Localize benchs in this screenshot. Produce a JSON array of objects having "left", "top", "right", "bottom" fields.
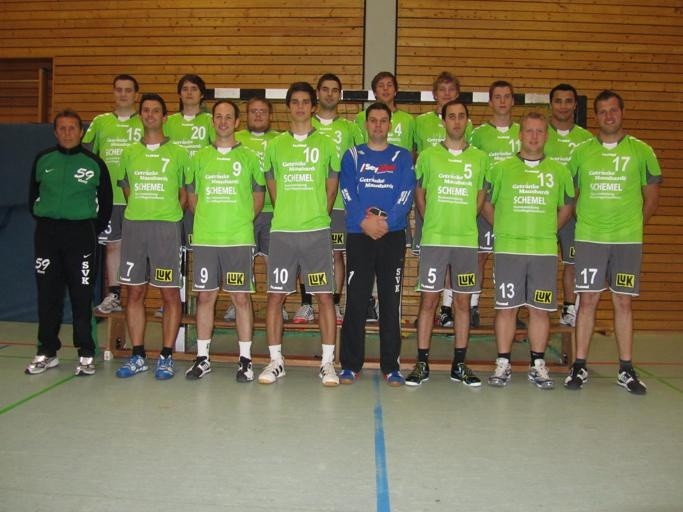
[{"left": 94, "top": 305, "right": 613, "bottom": 375}]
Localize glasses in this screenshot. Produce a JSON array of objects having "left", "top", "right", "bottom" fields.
[{"left": 248, "top": 108, "right": 269, "bottom": 116}]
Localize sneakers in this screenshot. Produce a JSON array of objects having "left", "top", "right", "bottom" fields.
[
  {"left": 338, "top": 369, "right": 361, "bottom": 384},
  {"left": 564, "top": 366, "right": 588, "bottom": 389},
  {"left": 258, "top": 356, "right": 286, "bottom": 383},
  {"left": 185, "top": 356, "right": 211, "bottom": 379},
  {"left": 293, "top": 305, "right": 314, "bottom": 324},
  {"left": 470, "top": 307, "right": 480, "bottom": 329},
  {"left": 405, "top": 360, "right": 429, "bottom": 386},
  {"left": 223, "top": 305, "right": 235, "bottom": 323},
  {"left": 451, "top": 363, "right": 482, "bottom": 386},
  {"left": 94, "top": 293, "right": 124, "bottom": 313},
  {"left": 25, "top": 353, "right": 59, "bottom": 374},
  {"left": 366, "top": 296, "right": 378, "bottom": 322},
  {"left": 155, "top": 354, "right": 174, "bottom": 379},
  {"left": 74, "top": 356, "right": 96, "bottom": 376},
  {"left": 236, "top": 357, "right": 254, "bottom": 382},
  {"left": 560, "top": 305, "right": 577, "bottom": 327},
  {"left": 335, "top": 304, "right": 344, "bottom": 325},
  {"left": 527, "top": 358, "right": 554, "bottom": 389},
  {"left": 318, "top": 362, "right": 339, "bottom": 387},
  {"left": 282, "top": 308, "right": 289, "bottom": 321},
  {"left": 116, "top": 354, "right": 148, "bottom": 378},
  {"left": 517, "top": 318, "right": 526, "bottom": 329},
  {"left": 154, "top": 306, "right": 164, "bottom": 318},
  {"left": 440, "top": 306, "right": 453, "bottom": 328},
  {"left": 616, "top": 369, "right": 647, "bottom": 394},
  {"left": 384, "top": 370, "right": 404, "bottom": 387},
  {"left": 487, "top": 358, "right": 511, "bottom": 386}
]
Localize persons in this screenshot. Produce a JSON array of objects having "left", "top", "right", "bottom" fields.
[
  {"left": 292, "top": 74, "right": 365, "bottom": 326},
  {"left": 540, "top": 82, "right": 595, "bottom": 328},
  {"left": 480, "top": 113, "right": 574, "bottom": 389},
  {"left": 466, "top": 79, "right": 527, "bottom": 335},
  {"left": 79, "top": 74, "right": 144, "bottom": 317},
  {"left": 413, "top": 70, "right": 474, "bottom": 330},
  {"left": 562, "top": 90, "right": 662, "bottom": 394},
  {"left": 223, "top": 96, "right": 289, "bottom": 322},
  {"left": 354, "top": 70, "right": 413, "bottom": 323},
  {"left": 115, "top": 92, "right": 191, "bottom": 380},
  {"left": 154, "top": 75, "right": 217, "bottom": 318},
  {"left": 403, "top": 101, "right": 491, "bottom": 388},
  {"left": 23, "top": 108, "right": 112, "bottom": 376},
  {"left": 256, "top": 81, "right": 340, "bottom": 387},
  {"left": 337, "top": 103, "right": 416, "bottom": 388},
  {"left": 185, "top": 99, "right": 266, "bottom": 382}
]
